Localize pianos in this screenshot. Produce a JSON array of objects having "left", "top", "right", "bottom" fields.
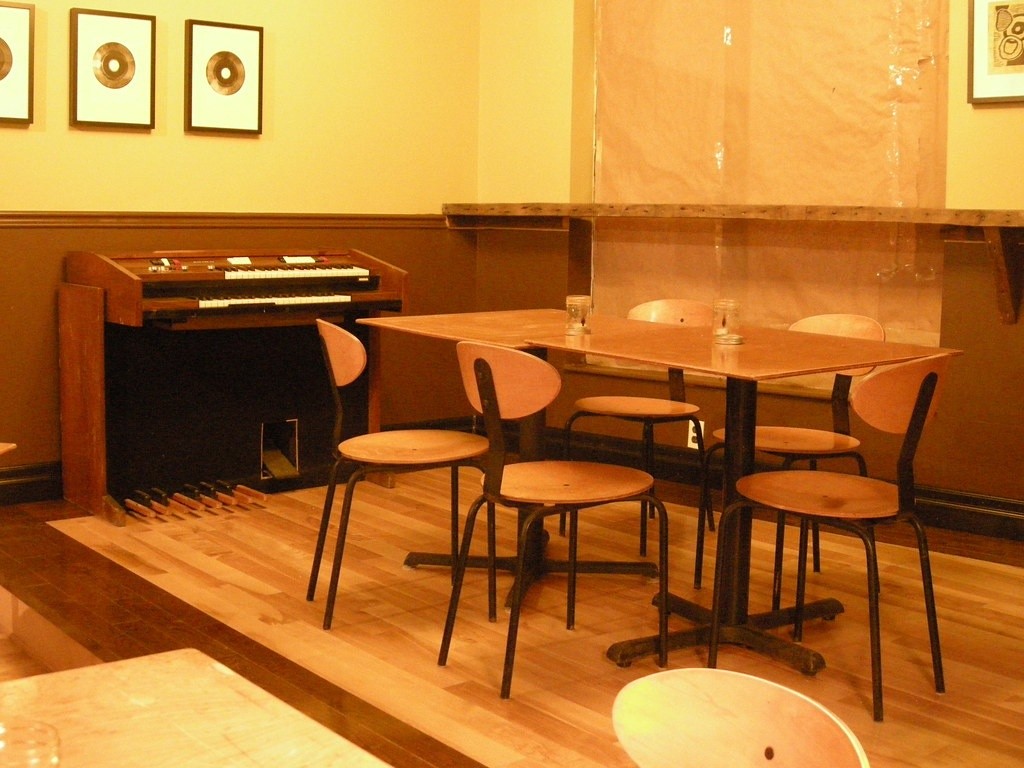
[{"left": 51, "top": 246, "right": 411, "bottom": 528}]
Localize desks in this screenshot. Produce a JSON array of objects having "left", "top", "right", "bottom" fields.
[
  {"left": 0, "top": 648, "right": 394, "bottom": 768},
  {"left": 359, "top": 308, "right": 968, "bottom": 676}
]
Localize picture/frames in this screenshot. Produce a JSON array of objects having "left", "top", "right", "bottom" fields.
[
  {"left": 0, "top": 1, "right": 36, "bottom": 124},
  {"left": 184, "top": 18, "right": 264, "bottom": 135},
  {"left": 69, "top": 8, "right": 157, "bottom": 129},
  {"left": 967, "top": 0, "right": 1024, "bottom": 103}
]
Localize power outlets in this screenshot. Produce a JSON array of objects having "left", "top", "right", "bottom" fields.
[{"left": 687, "top": 420, "right": 705, "bottom": 449}]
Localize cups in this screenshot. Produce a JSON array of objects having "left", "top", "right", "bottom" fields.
[
  {"left": 712, "top": 299, "right": 744, "bottom": 345},
  {"left": 564, "top": 295, "right": 591, "bottom": 336}
]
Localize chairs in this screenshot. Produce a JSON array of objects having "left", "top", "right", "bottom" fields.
[
  {"left": 611, "top": 668, "right": 871, "bottom": 768},
  {"left": 559, "top": 298, "right": 718, "bottom": 557},
  {"left": 437, "top": 341, "right": 670, "bottom": 699},
  {"left": 306, "top": 318, "right": 498, "bottom": 630},
  {"left": 694, "top": 313, "right": 886, "bottom": 612},
  {"left": 706, "top": 353, "right": 953, "bottom": 724}
]
